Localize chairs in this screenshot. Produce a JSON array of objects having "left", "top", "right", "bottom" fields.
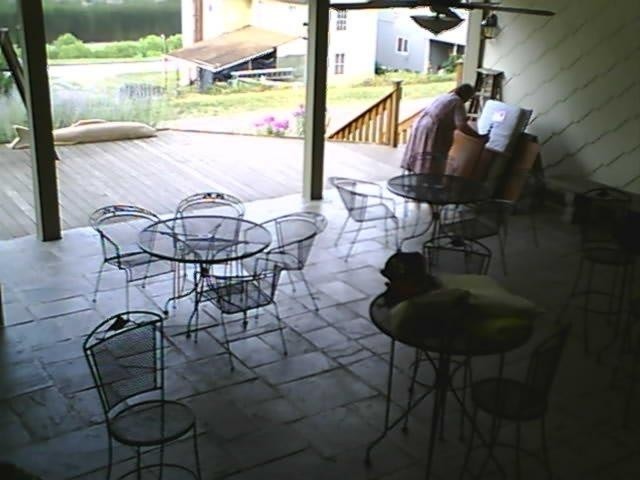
[
  {"left": 607, "top": 219, "right": 640, "bottom": 408},
  {"left": 422, "top": 235, "right": 493, "bottom": 276},
  {"left": 136, "top": 215, "right": 273, "bottom": 343},
  {"left": 83, "top": 310, "right": 202, "bottom": 479},
  {"left": 438, "top": 196, "right": 514, "bottom": 278},
  {"left": 471, "top": 319, "right": 574, "bottom": 478},
  {"left": 88, "top": 204, "right": 178, "bottom": 321},
  {"left": 170, "top": 191, "right": 246, "bottom": 311},
  {"left": 241, "top": 210, "right": 329, "bottom": 319},
  {"left": 194, "top": 257, "right": 290, "bottom": 374},
  {"left": 553, "top": 187, "right": 632, "bottom": 359},
  {"left": 327, "top": 176, "right": 399, "bottom": 264}
]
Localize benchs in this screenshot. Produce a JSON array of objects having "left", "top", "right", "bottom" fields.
[{"left": 545, "top": 176, "right": 639, "bottom": 226}]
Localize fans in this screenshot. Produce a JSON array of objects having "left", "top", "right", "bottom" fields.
[{"left": 329, "top": 0, "right": 555, "bottom": 35}]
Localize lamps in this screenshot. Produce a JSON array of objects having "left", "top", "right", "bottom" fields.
[{"left": 480, "top": 14, "right": 496, "bottom": 39}]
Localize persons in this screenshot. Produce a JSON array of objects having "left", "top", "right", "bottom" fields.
[{"left": 400, "top": 83, "right": 489, "bottom": 177}]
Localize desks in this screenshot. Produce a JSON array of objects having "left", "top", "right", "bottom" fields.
[
  {"left": 387, "top": 173, "right": 490, "bottom": 253},
  {"left": 365, "top": 276, "right": 538, "bottom": 478}
]
